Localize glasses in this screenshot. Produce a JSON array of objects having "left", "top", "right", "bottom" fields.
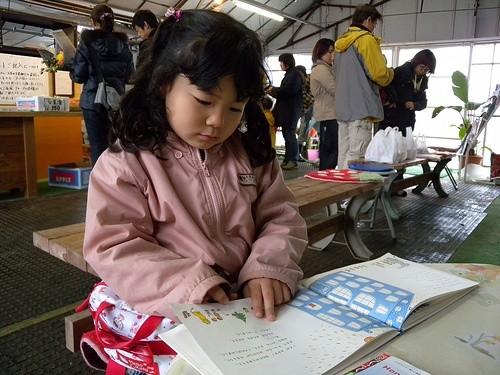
[{"left": 420, "top": 64, "right": 430, "bottom": 73}]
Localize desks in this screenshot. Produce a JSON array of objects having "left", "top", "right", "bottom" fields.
[
  {"left": 0, "top": 112, "right": 86, "bottom": 199},
  {"left": 161, "top": 263, "right": 500, "bottom": 375}
]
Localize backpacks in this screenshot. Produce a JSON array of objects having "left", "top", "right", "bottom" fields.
[{"left": 74, "top": 280, "right": 178, "bottom": 374}]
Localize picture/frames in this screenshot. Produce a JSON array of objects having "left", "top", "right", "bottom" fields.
[{"left": 52, "top": 67, "right": 74, "bottom": 98}]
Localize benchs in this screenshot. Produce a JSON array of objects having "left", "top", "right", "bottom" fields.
[
  {"left": 32, "top": 168, "right": 389, "bottom": 283},
  {"left": 348, "top": 147, "right": 457, "bottom": 198}
]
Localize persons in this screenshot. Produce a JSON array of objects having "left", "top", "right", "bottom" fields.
[
  {"left": 334, "top": 3, "right": 395, "bottom": 210},
  {"left": 125, "top": 10, "right": 160, "bottom": 82},
  {"left": 260, "top": 97, "right": 278, "bottom": 150},
  {"left": 378, "top": 48, "right": 436, "bottom": 197},
  {"left": 263, "top": 52, "right": 303, "bottom": 169},
  {"left": 310, "top": 38, "right": 339, "bottom": 172},
  {"left": 69, "top": 3, "right": 135, "bottom": 168},
  {"left": 73, "top": 8, "right": 310, "bottom": 375},
  {"left": 295, "top": 65, "right": 321, "bottom": 162}
]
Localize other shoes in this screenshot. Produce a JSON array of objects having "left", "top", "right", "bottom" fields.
[
  {"left": 283, "top": 160, "right": 299, "bottom": 169},
  {"left": 392, "top": 189, "right": 407, "bottom": 197},
  {"left": 298, "top": 154, "right": 307, "bottom": 162},
  {"left": 280, "top": 161, "right": 287, "bottom": 167}
]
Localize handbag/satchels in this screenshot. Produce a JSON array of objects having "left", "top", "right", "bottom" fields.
[{"left": 94, "top": 80, "right": 123, "bottom": 124}]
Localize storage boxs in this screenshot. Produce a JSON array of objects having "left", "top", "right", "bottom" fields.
[
  {"left": 47, "top": 164, "right": 93, "bottom": 190},
  {"left": 17, "top": 96, "right": 70, "bottom": 112}
]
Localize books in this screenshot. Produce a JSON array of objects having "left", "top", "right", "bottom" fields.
[{"left": 159, "top": 252, "right": 479, "bottom": 375}]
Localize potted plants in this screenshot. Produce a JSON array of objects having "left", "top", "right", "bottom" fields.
[{"left": 432, "top": 71, "right": 493, "bottom": 169}]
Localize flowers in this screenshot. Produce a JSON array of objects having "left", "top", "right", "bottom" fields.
[{"left": 37, "top": 48, "right": 65, "bottom": 75}]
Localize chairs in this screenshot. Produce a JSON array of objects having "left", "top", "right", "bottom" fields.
[{"left": 426, "top": 124, "right": 472, "bottom": 190}]
